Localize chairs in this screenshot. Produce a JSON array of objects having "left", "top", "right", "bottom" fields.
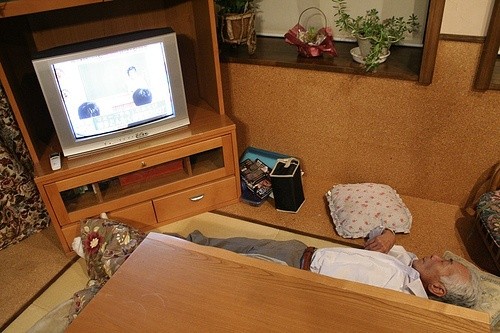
[{"left": 465, "top": 165, "right": 500, "bottom": 269}]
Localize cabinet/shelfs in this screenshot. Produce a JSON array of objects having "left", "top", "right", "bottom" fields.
[{"left": 0, "top": 0, "right": 241, "bottom": 256}]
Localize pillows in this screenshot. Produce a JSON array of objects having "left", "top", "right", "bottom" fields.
[
  {"left": 324, "top": 183, "right": 413, "bottom": 240},
  {"left": 441, "top": 251, "right": 500, "bottom": 327},
  {"left": 81, "top": 219, "right": 145, "bottom": 285}
]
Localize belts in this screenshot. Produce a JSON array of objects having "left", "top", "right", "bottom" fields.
[{"left": 300, "top": 246, "right": 316, "bottom": 271}]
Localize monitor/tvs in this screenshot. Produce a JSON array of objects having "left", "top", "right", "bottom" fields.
[{"left": 31, "top": 27, "right": 190, "bottom": 161}]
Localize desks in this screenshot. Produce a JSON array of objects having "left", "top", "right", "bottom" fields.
[{"left": 62, "top": 231, "right": 491, "bottom": 333}]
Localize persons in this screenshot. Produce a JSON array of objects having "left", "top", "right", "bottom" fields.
[
  {"left": 77, "top": 102, "right": 101, "bottom": 119},
  {"left": 128, "top": 67, "right": 141, "bottom": 88},
  {"left": 133, "top": 88, "right": 152, "bottom": 107},
  {"left": 72, "top": 212, "right": 481, "bottom": 310}
]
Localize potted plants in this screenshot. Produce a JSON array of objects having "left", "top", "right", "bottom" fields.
[
  {"left": 215, "top": 0, "right": 263, "bottom": 55},
  {"left": 333, "top": 0, "right": 422, "bottom": 72}
]
